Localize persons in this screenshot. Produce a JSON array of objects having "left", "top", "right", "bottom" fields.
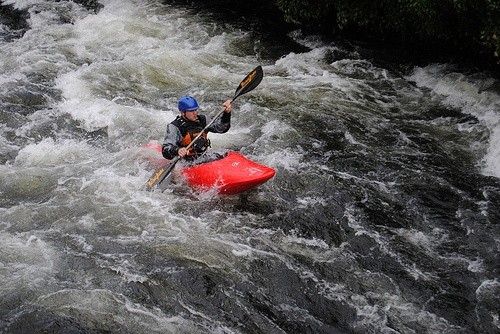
[{"left": 162, "top": 96, "right": 231, "bottom": 167}]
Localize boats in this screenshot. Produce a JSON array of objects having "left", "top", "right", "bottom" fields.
[{"left": 139, "top": 145, "right": 277, "bottom": 197}]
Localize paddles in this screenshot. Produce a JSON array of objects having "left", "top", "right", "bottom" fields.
[{"left": 146, "top": 65, "right": 264, "bottom": 192}]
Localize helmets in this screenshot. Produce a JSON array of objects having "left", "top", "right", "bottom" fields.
[{"left": 178, "top": 96, "right": 199, "bottom": 111}]
